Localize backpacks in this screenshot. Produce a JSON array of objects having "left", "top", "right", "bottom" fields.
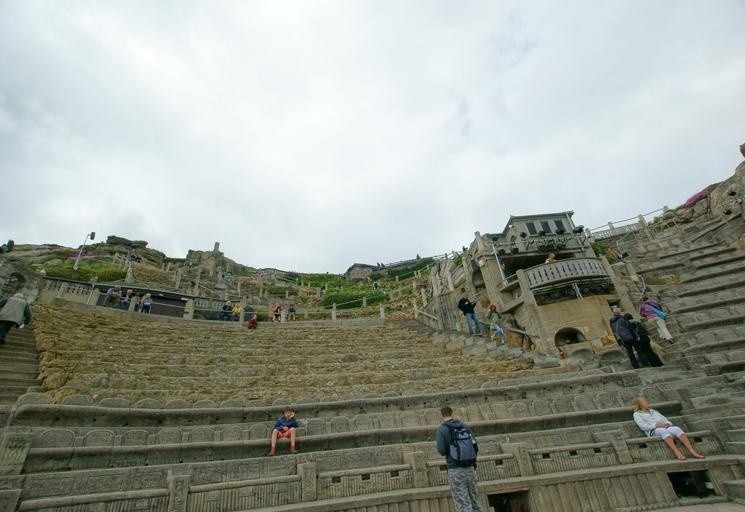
[{"left": 442, "top": 423, "right": 478, "bottom": 463}]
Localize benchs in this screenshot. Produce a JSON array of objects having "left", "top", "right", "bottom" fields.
[{"left": 7, "top": 302, "right": 745, "bottom": 512}]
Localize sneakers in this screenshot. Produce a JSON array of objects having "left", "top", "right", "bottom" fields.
[
  {"left": 268, "top": 448, "right": 275, "bottom": 455},
  {"left": 291, "top": 446, "right": 296, "bottom": 452}
]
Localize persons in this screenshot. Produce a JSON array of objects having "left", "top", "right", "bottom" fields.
[
  {"left": 609, "top": 307, "right": 650, "bottom": 369},
  {"left": 0, "top": 293, "right": 31, "bottom": 345},
  {"left": 119, "top": 288, "right": 132, "bottom": 310},
  {"left": 632, "top": 395, "right": 705, "bottom": 460},
  {"left": 268, "top": 406, "right": 299, "bottom": 456},
  {"left": 457, "top": 292, "right": 481, "bottom": 336},
  {"left": 624, "top": 312, "right": 664, "bottom": 368},
  {"left": 140, "top": 293, "right": 152, "bottom": 313},
  {"left": 486, "top": 304, "right": 505, "bottom": 343},
  {"left": 545, "top": 252, "right": 556, "bottom": 263},
  {"left": 436, "top": 407, "right": 487, "bottom": 512},
  {"left": 247, "top": 314, "right": 257, "bottom": 328},
  {"left": 271, "top": 302, "right": 282, "bottom": 322},
  {"left": 640, "top": 296, "right": 674, "bottom": 344},
  {"left": 101, "top": 285, "right": 122, "bottom": 308},
  {"left": 233, "top": 302, "right": 240, "bottom": 317},
  {"left": 288, "top": 303, "right": 296, "bottom": 320},
  {"left": 221, "top": 300, "right": 233, "bottom": 321}
]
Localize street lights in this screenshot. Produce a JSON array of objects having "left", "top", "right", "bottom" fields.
[{"left": 74, "top": 230, "right": 96, "bottom": 270}]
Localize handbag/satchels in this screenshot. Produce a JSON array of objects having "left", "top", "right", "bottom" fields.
[
  {"left": 646, "top": 304, "right": 668, "bottom": 319},
  {"left": 614, "top": 333, "right": 624, "bottom": 348}
]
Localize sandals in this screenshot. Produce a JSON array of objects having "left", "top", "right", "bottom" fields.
[{"left": 677, "top": 454, "right": 704, "bottom": 459}]
